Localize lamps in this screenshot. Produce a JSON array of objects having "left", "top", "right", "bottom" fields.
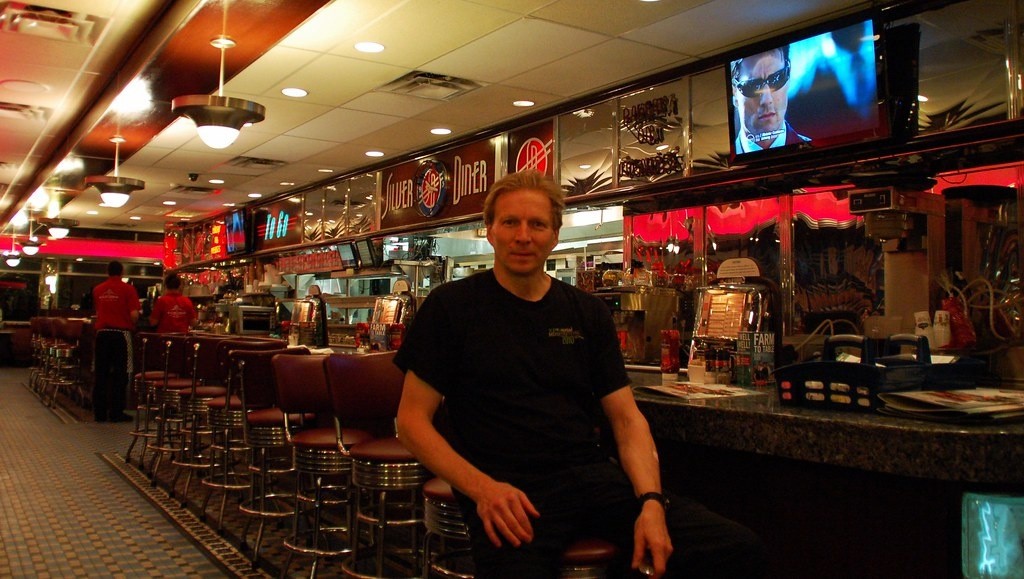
[
  {"left": 2, "top": 236, "right": 22, "bottom": 267},
  {"left": 171, "top": 0, "right": 266, "bottom": 150},
  {"left": 84, "top": 138, "right": 144, "bottom": 208},
  {"left": 37, "top": 190, "right": 79, "bottom": 238},
  {"left": 20, "top": 218, "right": 44, "bottom": 255}
]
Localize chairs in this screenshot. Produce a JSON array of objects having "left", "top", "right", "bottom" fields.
[{"left": 771, "top": 333, "right": 984, "bottom": 414}]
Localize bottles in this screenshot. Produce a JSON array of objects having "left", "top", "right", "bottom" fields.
[{"left": 660, "top": 329, "right": 680, "bottom": 386}]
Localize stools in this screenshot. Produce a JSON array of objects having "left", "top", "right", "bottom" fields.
[{"left": 28, "top": 317, "right": 665, "bottom": 579}]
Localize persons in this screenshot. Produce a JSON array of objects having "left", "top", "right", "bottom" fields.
[
  {"left": 148, "top": 273, "right": 197, "bottom": 335},
  {"left": 730, "top": 44, "right": 811, "bottom": 155},
  {"left": 393, "top": 171, "right": 764, "bottom": 579},
  {"left": 92, "top": 261, "right": 141, "bottom": 422}
]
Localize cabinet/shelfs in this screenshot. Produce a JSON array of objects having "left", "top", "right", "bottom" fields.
[{"left": 318, "top": 269, "right": 394, "bottom": 349}]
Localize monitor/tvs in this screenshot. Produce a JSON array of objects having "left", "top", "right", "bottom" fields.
[
  {"left": 355, "top": 238, "right": 376, "bottom": 267},
  {"left": 338, "top": 241, "right": 358, "bottom": 268},
  {"left": 224, "top": 208, "right": 248, "bottom": 256},
  {"left": 724, "top": 6, "right": 891, "bottom": 169}
]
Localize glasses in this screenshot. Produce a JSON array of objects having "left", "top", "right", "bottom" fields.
[{"left": 732, "top": 59, "right": 791, "bottom": 98}]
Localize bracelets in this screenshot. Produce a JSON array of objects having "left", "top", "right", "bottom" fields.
[{"left": 638, "top": 492, "right": 671, "bottom": 511}]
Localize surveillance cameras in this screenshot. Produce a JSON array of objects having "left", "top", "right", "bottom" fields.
[{"left": 189, "top": 173, "right": 198, "bottom": 181}]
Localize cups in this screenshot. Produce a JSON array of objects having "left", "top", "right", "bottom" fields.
[
  {"left": 914, "top": 310, "right": 952, "bottom": 350},
  {"left": 688, "top": 364, "right": 704, "bottom": 383}
]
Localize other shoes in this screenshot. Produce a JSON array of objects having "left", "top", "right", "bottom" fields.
[
  {"left": 94, "top": 412, "right": 108, "bottom": 420},
  {"left": 109, "top": 410, "right": 134, "bottom": 423}
]
